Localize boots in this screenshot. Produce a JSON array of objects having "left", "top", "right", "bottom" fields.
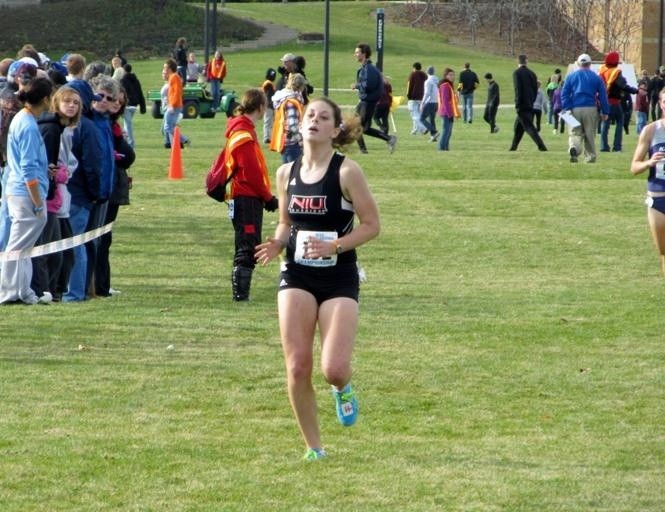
[{"left": 232, "top": 266, "right": 252, "bottom": 302}]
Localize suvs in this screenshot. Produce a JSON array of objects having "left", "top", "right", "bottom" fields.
[{"left": 147, "top": 80, "right": 248, "bottom": 118}]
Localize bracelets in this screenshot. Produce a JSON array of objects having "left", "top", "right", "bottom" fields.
[{"left": 332, "top": 238, "right": 343, "bottom": 255}]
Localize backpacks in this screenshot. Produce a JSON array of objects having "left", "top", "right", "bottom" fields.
[{"left": 207, "top": 147, "right": 238, "bottom": 202}]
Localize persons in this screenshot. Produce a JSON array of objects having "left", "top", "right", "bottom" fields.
[
  {"left": 631, "top": 88, "right": 665, "bottom": 275},
  {"left": 545, "top": 52, "right": 640, "bottom": 163},
  {"left": 252, "top": 98, "right": 381, "bottom": 466},
  {"left": 224, "top": 89, "right": 279, "bottom": 300},
  {"left": 635, "top": 65, "right": 665, "bottom": 136},
  {"left": 456, "top": 62, "right": 501, "bottom": 134},
  {"left": 160, "top": 35, "right": 314, "bottom": 164},
  {"left": 507, "top": 55, "right": 549, "bottom": 152},
  {"left": 407, "top": 62, "right": 458, "bottom": 151},
  {"left": 352, "top": 44, "right": 397, "bottom": 154},
  {"left": 1, "top": 42, "right": 146, "bottom": 306}
]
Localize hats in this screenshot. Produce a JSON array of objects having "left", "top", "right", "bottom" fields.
[
  {"left": 578, "top": 54, "right": 592, "bottom": 64},
  {"left": 605, "top": 52, "right": 619, "bottom": 65},
  {"left": 7, "top": 52, "right": 112, "bottom": 101},
  {"left": 427, "top": 66, "right": 433, "bottom": 75},
  {"left": 280, "top": 53, "right": 295, "bottom": 61}
]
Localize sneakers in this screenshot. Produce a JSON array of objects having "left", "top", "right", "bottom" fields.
[
  {"left": 165, "top": 141, "right": 187, "bottom": 148},
  {"left": 303, "top": 448, "right": 327, "bottom": 460},
  {"left": 411, "top": 129, "right": 440, "bottom": 143},
  {"left": 333, "top": 384, "right": 358, "bottom": 426},
  {"left": 570, "top": 148, "right": 578, "bottom": 162},
  {"left": 39, "top": 288, "right": 122, "bottom": 303},
  {"left": 265, "top": 139, "right": 271, "bottom": 143},
  {"left": 388, "top": 135, "right": 397, "bottom": 154},
  {"left": 211, "top": 107, "right": 221, "bottom": 112},
  {"left": 360, "top": 150, "right": 368, "bottom": 155}
]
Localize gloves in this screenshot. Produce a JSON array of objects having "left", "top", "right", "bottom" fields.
[{"left": 265, "top": 196, "right": 278, "bottom": 212}]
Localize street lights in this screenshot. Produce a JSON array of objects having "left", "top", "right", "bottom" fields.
[{"left": 376, "top": 7, "right": 385, "bottom": 73}]
